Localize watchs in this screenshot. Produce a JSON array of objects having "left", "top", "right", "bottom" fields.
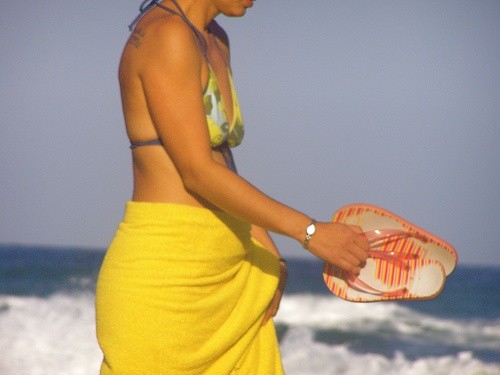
[{"left": 303, "top": 217, "right": 317, "bottom": 250}]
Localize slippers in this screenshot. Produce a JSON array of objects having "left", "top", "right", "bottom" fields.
[
  {"left": 330, "top": 204, "right": 458, "bottom": 276},
  {"left": 323, "top": 249, "right": 447, "bottom": 303}
]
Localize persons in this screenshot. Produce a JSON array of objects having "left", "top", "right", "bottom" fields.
[{"left": 95, "top": 0, "right": 368, "bottom": 375}]
